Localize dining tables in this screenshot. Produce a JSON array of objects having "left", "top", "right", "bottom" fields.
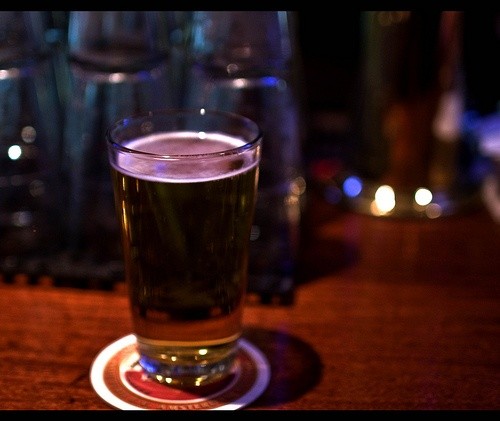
[{"left": 0, "top": 111, "right": 500, "bottom": 412}]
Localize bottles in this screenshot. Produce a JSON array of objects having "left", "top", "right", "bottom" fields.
[
  {"left": 180, "top": 8, "right": 305, "bottom": 303},
  {"left": 57, "top": 13, "right": 183, "bottom": 288},
  {"left": 3, "top": 12, "right": 71, "bottom": 288}
]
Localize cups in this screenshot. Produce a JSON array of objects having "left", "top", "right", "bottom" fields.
[
  {"left": 108, "top": 105, "right": 264, "bottom": 391},
  {"left": 325, "top": 11, "right": 486, "bottom": 223}
]
